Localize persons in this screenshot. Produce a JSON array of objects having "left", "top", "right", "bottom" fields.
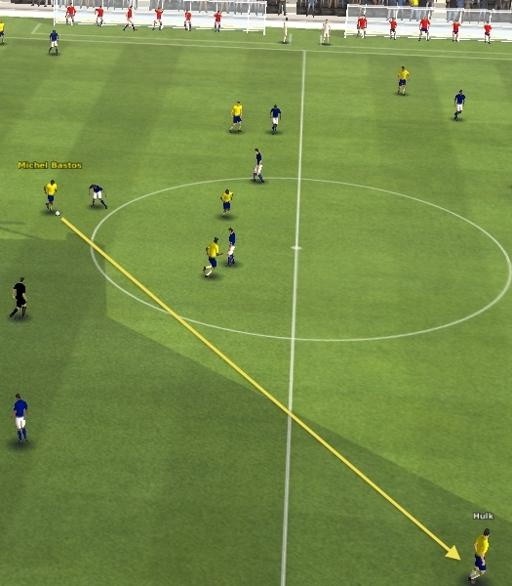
[
  {"left": 469, "top": 528, "right": 489, "bottom": 584},
  {"left": 12, "top": 393, "right": 28, "bottom": 443},
  {"left": 10, "top": 276, "right": 29, "bottom": 320},
  {"left": 1, "top": 0, "right": 512, "bottom": 586}
]
